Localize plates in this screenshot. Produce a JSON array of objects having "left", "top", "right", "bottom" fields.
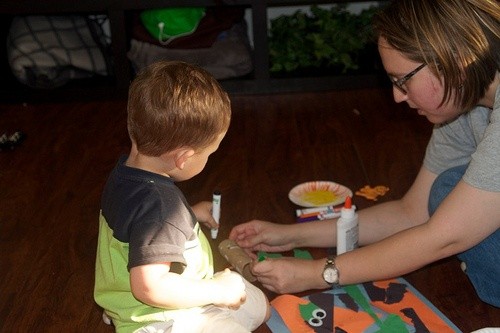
[{"left": 288, "top": 181, "right": 354, "bottom": 207}]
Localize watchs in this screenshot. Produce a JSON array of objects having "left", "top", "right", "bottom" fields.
[{"left": 321, "top": 255, "right": 342, "bottom": 289}]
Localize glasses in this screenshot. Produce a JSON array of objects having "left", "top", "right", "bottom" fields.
[{"left": 392, "top": 59, "right": 431, "bottom": 95}]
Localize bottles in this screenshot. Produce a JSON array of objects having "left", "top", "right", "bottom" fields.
[{"left": 336, "top": 196, "right": 360, "bottom": 256}]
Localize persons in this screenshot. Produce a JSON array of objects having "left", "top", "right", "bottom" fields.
[
  {"left": 92, "top": 59, "right": 272, "bottom": 333},
  {"left": 227, "top": 0, "right": 500, "bottom": 308}
]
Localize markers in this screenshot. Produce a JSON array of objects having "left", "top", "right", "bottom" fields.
[
  {"left": 285, "top": 201, "right": 359, "bottom": 226},
  {"left": 211, "top": 190, "right": 221, "bottom": 240}
]
